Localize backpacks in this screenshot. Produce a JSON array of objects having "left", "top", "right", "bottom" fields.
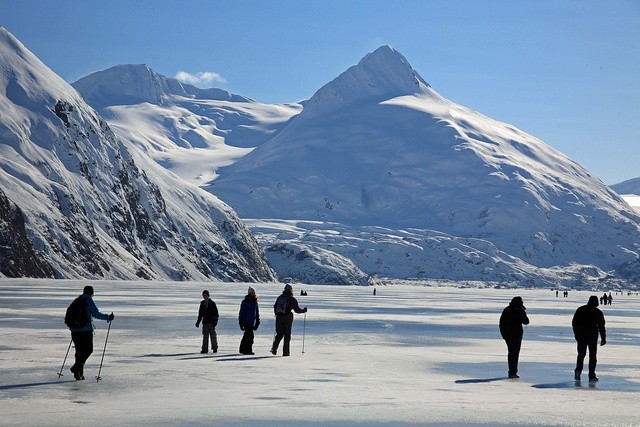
[
  {"left": 276, "top": 296, "right": 291, "bottom": 315},
  {"left": 65, "top": 295, "right": 90, "bottom": 330}
]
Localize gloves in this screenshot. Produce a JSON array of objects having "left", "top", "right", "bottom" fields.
[
  {"left": 109, "top": 313, "right": 114, "bottom": 320},
  {"left": 213, "top": 318, "right": 217, "bottom": 325},
  {"left": 239, "top": 321, "right": 244, "bottom": 331},
  {"left": 195, "top": 320, "right": 200, "bottom": 327},
  {"left": 303, "top": 307, "right": 307, "bottom": 312},
  {"left": 600, "top": 338, "right": 606, "bottom": 345},
  {"left": 253, "top": 322, "right": 260, "bottom": 330}
]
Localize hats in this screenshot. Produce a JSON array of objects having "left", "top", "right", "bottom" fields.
[
  {"left": 285, "top": 284, "right": 293, "bottom": 292},
  {"left": 203, "top": 290, "right": 209, "bottom": 297},
  {"left": 248, "top": 287, "right": 255, "bottom": 294},
  {"left": 85, "top": 286, "right": 94, "bottom": 295},
  {"left": 588, "top": 296, "right": 598, "bottom": 306}
]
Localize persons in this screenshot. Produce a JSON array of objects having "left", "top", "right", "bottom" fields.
[
  {"left": 498, "top": 296, "right": 530, "bottom": 378},
  {"left": 563, "top": 290, "right": 568, "bottom": 297},
  {"left": 600, "top": 292, "right": 614, "bottom": 305},
  {"left": 238, "top": 287, "right": 260, "bottom": 355},
  {"left": 195, "top": 290, "right": 219, "bottom": 353},
  {"left": 270, "top": 284, "right": 307, "bottom": 356},
  {"left": 571, "top": 296, "right": 607, "bottom": 381},
  {"left": 64, "top": 285, "right": 114, "bottom": 379},
  {"left": 556, "top": 290, "right": 559, "bottom": 296}
]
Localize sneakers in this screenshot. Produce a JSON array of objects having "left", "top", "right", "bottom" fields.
[
  {"left": 70, "top": 365, "right": 81, "bottom": 380},
  {"left": 213, "top": 349, "right": 217, "bottom": 353},
  {"left": 509, "top": 374, "right": 519, "bottom": 378},
  {"left": 271, "top": 348, "right": 277, "bottom": 355},
  {"left": 201, "top": 350, "right": 208, "bottom": 353},
  {"left": 80, "top": 375, "right": 84, "bottom": 379},
  {"left": 575, "top": 370, "right": 581, "bottom": 380},
  {"left": 590, "top": 376, "right": 598, "bottom": 381},
  {"left": 243, "top": 351, "right": 254, "bottom": 355}
]
